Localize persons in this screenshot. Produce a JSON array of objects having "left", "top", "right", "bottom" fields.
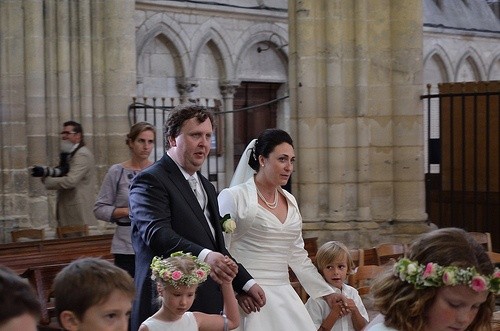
[
  {"left": 213, "top": 128, "right": 352, "bottom": 330},
  {"left": 300, "top": 238, "right": 369, "bottom": 331},
  {"left": 126, "top": 105, "right": 266, "bottom": 330},
  {"left": 39, "top": 121, "right": 99, "bottom": 240},
  {"left": 51, "top": 253, "right": 139, "bottom": 331},
  {"left": 93, "top": 120, "right": 157, "bottom": 284},
  {"left": 136, "top": 250, "right": 242, "bottom": 331},
  {"left": 361, "top": 224, "right": 500, "bottom": 331},
  {"left": 0, "top": 260, "right": 44, "bottom": 331}
]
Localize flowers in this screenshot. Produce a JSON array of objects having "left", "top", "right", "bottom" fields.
[
  {"left": 149, "top": 249, "right": 213, "bottom": 289},
  {"left": 394, "top": 257, "right": 499, "bottom": 294},
  {"left": 222, "top": 214, "right": 237, "bottom": 234}
]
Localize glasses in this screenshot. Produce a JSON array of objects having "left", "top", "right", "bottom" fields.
[{"left": 61, "top": 131, "right": 76, "bottom": 135}]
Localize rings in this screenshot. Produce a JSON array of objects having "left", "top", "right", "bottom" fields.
[{"left": 247, "top": 306, "right": 251, "bottom": 309}]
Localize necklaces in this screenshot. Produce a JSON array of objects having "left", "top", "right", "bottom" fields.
[{"left": 252, "top": 177, "right": 281, "bottom": 211}]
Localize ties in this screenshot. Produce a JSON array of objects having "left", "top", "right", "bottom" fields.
[{"left": 188, "top": 177, "right": 198, "bottom": 197}]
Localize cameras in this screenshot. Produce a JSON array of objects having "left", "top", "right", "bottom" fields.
[{"left": 32, "top": 165, "right": 67, "bottom": 177}]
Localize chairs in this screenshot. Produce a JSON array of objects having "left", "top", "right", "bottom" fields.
[
  {"left": 11, "top": 227, "right": 44, "bottom": 242},
  {"left": 290, "top": 232, "right": 500, "bottom": 314},
  {"left": 56, "top": 223, "right": 89, "bottom": 238}
]
